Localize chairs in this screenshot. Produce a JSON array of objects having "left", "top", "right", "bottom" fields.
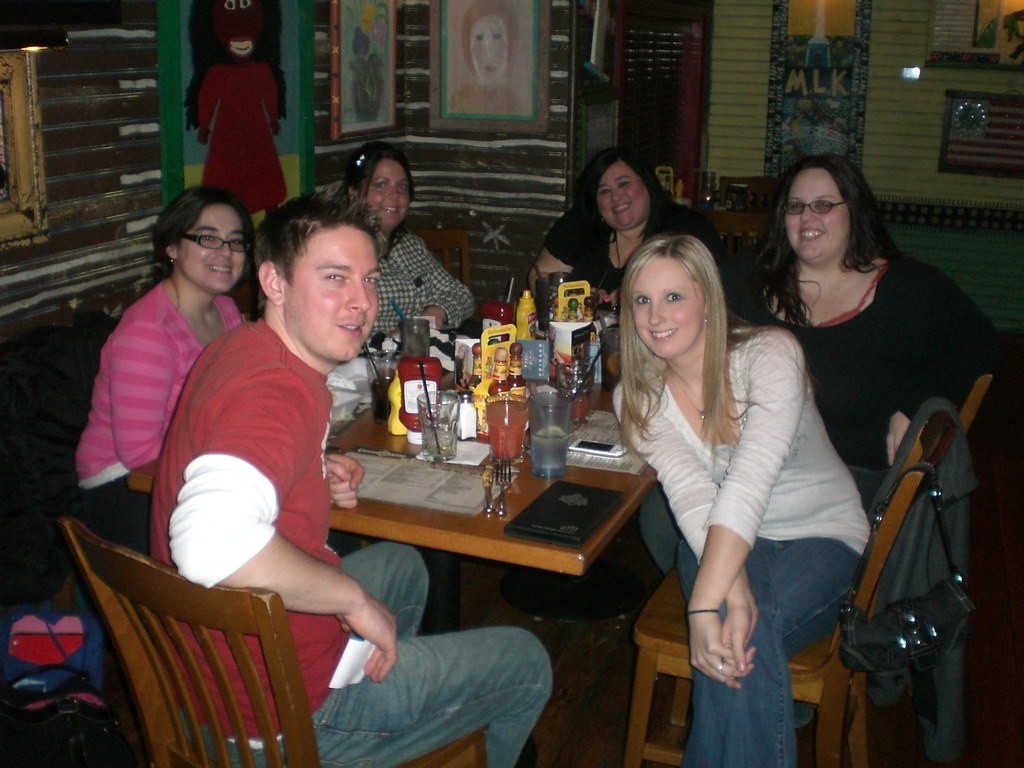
[
  {"left": 627, "top": 373, "right": 993, "bottom": 768},
  {"left": 410, "top": 228, "right": 471, "bottom": 292},
  {"left": 61, "top": 514, "right": 490, "bottom": 768},
  {"left": 695, "top": 176, "right": 782, "bottom": 263}
]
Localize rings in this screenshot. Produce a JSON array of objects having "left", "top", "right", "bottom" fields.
[{"left": 716, "top": 658, "right": 727, "bottom": 671}]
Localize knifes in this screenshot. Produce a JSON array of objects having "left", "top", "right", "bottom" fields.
[{"left": 482, "top": 465, "right": 494, "bottom": 513}]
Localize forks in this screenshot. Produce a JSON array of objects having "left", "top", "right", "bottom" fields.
[{"left": 496, "top": 459, "right": 511, "bottom": 519}]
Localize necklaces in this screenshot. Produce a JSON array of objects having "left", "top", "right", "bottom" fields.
[
  {"left": 611, "top": 229, "right": 643, "bottom": 267},
  {"left": 802, "top": 266, "right": 853, "bottom": 327},
  {"left": 667, "top": 365, "right": 706, "bottom": 421}
]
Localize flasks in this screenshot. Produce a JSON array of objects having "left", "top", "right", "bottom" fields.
[{"left": 542, "top": 272, "right": 571, "bottom": 332}]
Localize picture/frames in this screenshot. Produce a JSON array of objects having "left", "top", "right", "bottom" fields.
[
  {"left": 429, "top": 0, "right": 550, "bottom": 131},
  {"left": 925, "top": 0, "right": 1024, "bottom": 72},
  {"left": 155, "top": 0, "right": 315, "bottom": 231},
  {"left": 0, "top": 49, "right": 50, "bottom": 250},
  {"left": 937, "top": 89, "right": 1024, "bottom": 179},
  {"left": 330, "top": 0, "right": 397, "bottom": 141}
]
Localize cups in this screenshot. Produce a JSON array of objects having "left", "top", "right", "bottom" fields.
[
  {"left": 398, "top": 318, "right": 431, "bottom": 358},
  {"left": 417, "top": 391, "right": 461, "bottom": 462},
  {"left": 486, "top": 393, "right": 529, "bottom": 467},
  {"left": 529, "top": 391, "right": 573, "bottom": 479},
  {"left": 555, "top": 362, "right": 595, "bottom": 426},
  {"left": 367, "top": 350, "right": 403, "bottom": 425},
  {"left": 480, "top": 299, "right": 514, "bottom": 345},
  {"left": 599, "top": 327, "right": 621, "bottom": 390}
]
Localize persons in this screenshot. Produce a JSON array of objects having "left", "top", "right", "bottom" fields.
[
  {"left": 528, "top": 148, "right": 723, "bottom": 328},
  {"left": 637, "top": 149, "right": 1003, "bottom": 575},
  {"left": 74, "top": 184, "right": 363, "bottom": 558},
  {"left": 611, "top": 237, "right": 869, "bottom": 768},
  {"left": 150, "top": 205, "right": 553, "bottom": 768},
  {"left": 340, "top": 143, "right": 478, "bottom": 354}
]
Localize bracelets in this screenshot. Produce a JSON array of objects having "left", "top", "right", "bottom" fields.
[{"left": 685, "top": 608, "right": 721, "bottom": 614}]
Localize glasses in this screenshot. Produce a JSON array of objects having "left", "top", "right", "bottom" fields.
[
  {"left": 181, "top": 233, "right": 250, "bottom": 252},
  {"left": 781, "top": 199, "right": 850, "bottom": 216}
]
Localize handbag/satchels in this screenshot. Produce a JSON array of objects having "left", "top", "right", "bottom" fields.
[
  {"left": 0, "top": 605, "right": 108, "bottom": 716},
  {"left": 841, "top": 462, "right": 973, "bottom": 681}
]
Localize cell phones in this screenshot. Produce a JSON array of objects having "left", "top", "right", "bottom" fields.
[{"left": 568, "top": 439, "right": 628, "bottom": 457}]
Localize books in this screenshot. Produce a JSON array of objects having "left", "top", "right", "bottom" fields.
[{"left": 503, "top": 481, "right": 624, "bottom": 548}]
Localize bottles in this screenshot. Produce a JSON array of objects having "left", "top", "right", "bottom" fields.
[
  {"left": 488, "top": 347, "right": 510, "bottom": 396},
  {"left": 516, "top": 289, "right": 537, "bottom": 341},
  {"left": 387, "top": 370, "right": 408, "bottom": 436},
  {"left": 456, "top": 390, "right": 477, "bottom": 440},
  {"left": 399, "top": 356, "right": 442, "bottom": 445},
  {"left": 506, "top": 342, "right": 526, "bottom": 396},
  {"left": 467, "top": 343, "right": 482, "bottom": 392}
]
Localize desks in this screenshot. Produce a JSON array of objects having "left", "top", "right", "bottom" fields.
[{"left": 127, "top": 387, "right": 659, "bottom": 576}]
